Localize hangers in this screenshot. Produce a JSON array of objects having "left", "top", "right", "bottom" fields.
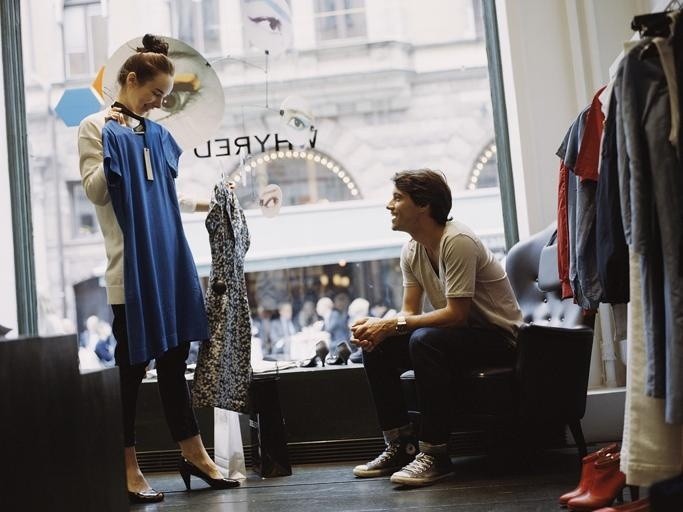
[
  {"left": 101, "top": 85, "right": 146, "bottom": 136},
  {"left": 630, "top": 0, "right": 683, "bottom": 38}
]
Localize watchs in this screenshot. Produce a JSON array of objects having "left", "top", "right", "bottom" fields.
[{"left": 395, "top": 316, "right": 407, "bottom": 336}]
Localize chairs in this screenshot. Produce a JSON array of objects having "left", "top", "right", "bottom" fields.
[{"left": 396, "top": 211, "right": 600, "bottom": 473}]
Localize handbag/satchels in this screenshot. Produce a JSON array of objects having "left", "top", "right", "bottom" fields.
[
  {"left": 248, "top": 366, "right": 292, "bottom": 478},
  {"left": 213, "top": 407, "right": 258, "bottom": 486}
]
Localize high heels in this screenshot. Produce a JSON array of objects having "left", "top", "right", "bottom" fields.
[
  {"left": 178, "top": 456, "right": 240, "bottom": 489},
  {"left": 299, "top": 340, "right": 329, "bottom": 367},
  {"left": 559, "top": 443, "right": 624, "bottom": 507},
  {"left": 127, "top": 488, "right": 164, "bottom": 504},
  {"left": 327, "top": 342, "right": 351, "bottom": 365},
  {"left": 567, "top": 453, "right": 641, "bottom": 510}
]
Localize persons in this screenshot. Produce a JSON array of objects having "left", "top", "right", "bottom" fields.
[
  {"left": 78, "top": 314, "right": 113, "bottom": 366},
  {"left": 251, "top": 297, "right": 388, "bottom": 365},
  {"left": 74, "top": 34, "right": 240, "bottom": 502},
  {"left": 349, "top": 167, "right": 527, "bottom": 487}
]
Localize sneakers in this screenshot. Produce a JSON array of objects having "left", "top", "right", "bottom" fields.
[
  {"left": 352, "top": 424, "right": 418, "bottom": 477},
  {"left": 390, "top": 441, "right": 456, "bottom": 487}
]
